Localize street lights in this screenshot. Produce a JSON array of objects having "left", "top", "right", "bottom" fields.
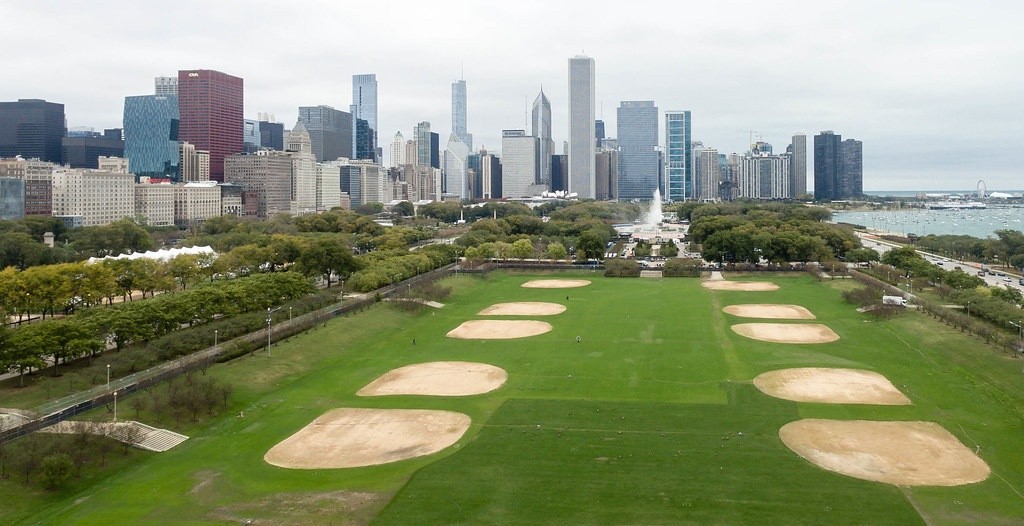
[
  {"left": 112, "top": 388, "right": 118, "bottom": 422},
  {"left": 289, "top": 306, "right": 293, "bottom": 323},
  {"left": 214, "top": 330, "right": 217, "bottom": 350},
  {"left": 888, "top": 271, "right": 890, "bottom": 282},
  {"left": 266, "top": 318, "right": 271, "bottom": 358},
  {"left": 340, "top": 291, "right": 343, "bottom": 306},
  {"left": 832, "top": 264, "right": 835, "bottom": 276},
  {"left": 899, "top": 275, "right": 909, "bottom": 292},
  {"left": 341, "top": 280, "right": 344, "bottom": 292},
  {"left": 967, "top": 300, "right": 970, "bottom": 319},
  {"left": 107, "top": 364, "right": 111, "bottom": 389}
]
